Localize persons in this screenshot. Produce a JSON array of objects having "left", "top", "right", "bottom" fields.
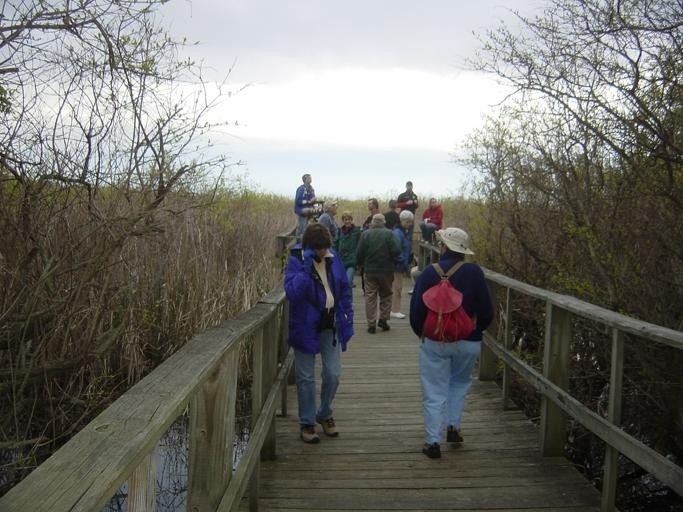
[
  {"left": 283, "top": 223, "right": 353, "bottom": 444},
  {"left": 419, "top": 197, "right": 443, "bottom": 244},
  {"left": 294, "top": 174, "right": 317, "bottom": 236},
  {"left": 317, "top": 198, "right": 415, "bottom": 333},
  {"left": 408, "top": 227, "right": 494, "bottom": 459},
  {"left": 396, "top": 181, "right": 418, "bottom": 215}
]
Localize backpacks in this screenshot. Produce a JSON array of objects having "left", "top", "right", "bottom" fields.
[{"left": 420, "top": 261, "right": 475, "bottom": 343}]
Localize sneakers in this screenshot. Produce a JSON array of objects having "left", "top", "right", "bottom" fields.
[
  {"left": 368, "top": 325, "right": 376, "bottom": 334},
  {"left": 316, "top": 410, "right": 339, "bottom": 436},
  {"left": 378, "top": 319, "right": 389, "bottom": 330},
  {"left": 423, "top": 442, "right": 442, "bottom": 458},
  {"left": 299, "top": 426, "right": 319, "bottom": 443},
  {"left": 390, "top": 311, "right": 406, "bottom": 319},
  {"left": 446, "top": 426, "right": 464, "bottom": 442}
]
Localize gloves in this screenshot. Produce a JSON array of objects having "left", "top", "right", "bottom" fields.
[{"left": 304, "top": 250, "right": 315, "bottom": 272}]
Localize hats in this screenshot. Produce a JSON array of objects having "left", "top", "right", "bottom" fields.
[
  {"left": 371, "top": 214, "right": 386, "bottom": 224},
  {"left": 324, "top": 200, "right": 337, "bottom": 212},
  {"left": 435, "top": 227, "right": 474, "bottom": 255}
]
